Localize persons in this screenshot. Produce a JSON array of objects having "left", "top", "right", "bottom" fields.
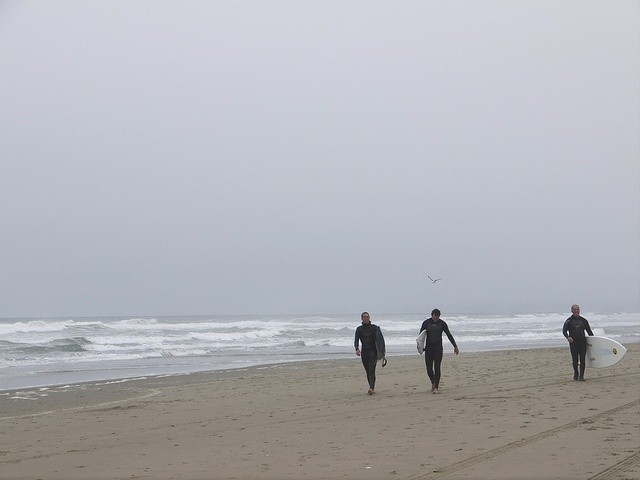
[
  {"left": 354, "top": 312, "right": 385, "bottom": 395},
  {"left": 563, "top": 304, "right": 593, "bottom": 381},
  {"left": 418, "top": 308, "right": 459, "bottom": 394}
]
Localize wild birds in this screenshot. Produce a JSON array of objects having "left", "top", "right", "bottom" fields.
[{"left": 428, "top": 275, "right": 441, "bottom": 283}]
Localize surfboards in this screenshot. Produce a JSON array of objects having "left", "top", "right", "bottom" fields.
[
  {"left": 578, "top": 336, "right": 627, "bottom": 369},
  {"left": 375, "top": 326, "right": 385, "bottom": 360},
  {"left": 416, "top": 328, "right": 426, "bottom": 354}
]
